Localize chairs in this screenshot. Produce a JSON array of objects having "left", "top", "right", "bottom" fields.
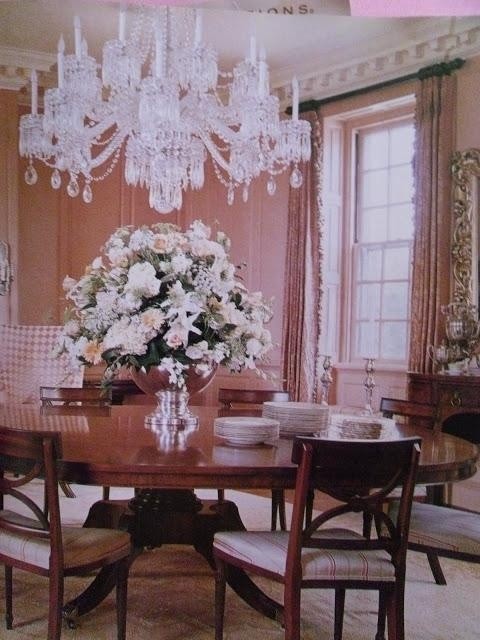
[
  {"left": 0, "top": 387, "right": 480, "bottom": 640},
  {"left": 0, "top": 322, "right": 91, "bottom": 500}
]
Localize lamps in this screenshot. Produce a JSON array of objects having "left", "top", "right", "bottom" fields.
[{"left": 17, "top": 1, "right": 313, "bottom": 216}]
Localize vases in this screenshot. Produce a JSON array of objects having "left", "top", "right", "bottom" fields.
[
  {"left": 129, "top": 354, "right": 224, "bottom": 431},
  {"left": 443, "top": 300, "right": 476, "bottom": 362}
]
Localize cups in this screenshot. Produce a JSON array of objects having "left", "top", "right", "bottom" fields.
[{"left": 447, "top": 363, "right": 465, "bottom": 370}]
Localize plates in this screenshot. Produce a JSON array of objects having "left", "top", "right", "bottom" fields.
[
  {"left": 335, "top": 415, "right": 396, "bottom": 449},
  {"left": 260, "top": 399, "right": 332, "bottom": 441},
  {"left": 445, "top": 370, "right": 467, "bottom": 375},
  {"left": 212, "top": 415, "right": 280, "bottom": 449}
]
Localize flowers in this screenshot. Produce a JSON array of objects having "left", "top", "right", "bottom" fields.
[{"left": 49, "top": 218, "right": 285, "bottom": 393}]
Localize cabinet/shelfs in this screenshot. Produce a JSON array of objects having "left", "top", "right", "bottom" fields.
[{"left": 404, "top": 368, "right": 480, "bottom": 435}]
